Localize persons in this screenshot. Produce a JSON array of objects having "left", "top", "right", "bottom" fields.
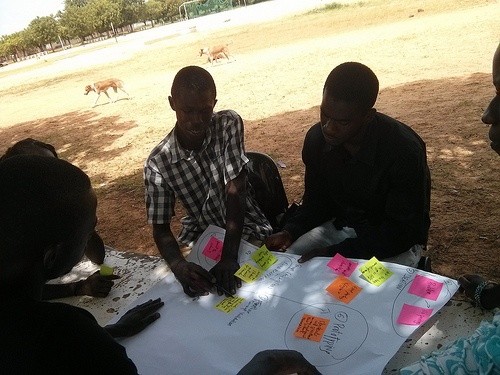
[
  {"left": 142, "top": 66, "right": 288, "bottom": 297},
  {"left": 258, "top": 63, "right": 430, "bottom": 269},
  {"left": 0, "top": 140, "right": 321, "bottom": 375},
  {"left": 398, "top": 38, "right": 500, "bottom": 375}
]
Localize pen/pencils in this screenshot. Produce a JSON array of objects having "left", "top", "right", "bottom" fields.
[{"left": 216, "top": 284, "right": 235, "bottom": 299}]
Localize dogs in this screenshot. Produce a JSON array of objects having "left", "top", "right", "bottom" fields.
[
  {"left": 199, "top": 41, "right": 233, "bottom": 66},
  {"left": 84, "top": 77, "right": 131, "bottom": 108}
]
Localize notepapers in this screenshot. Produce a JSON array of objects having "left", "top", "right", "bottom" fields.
[{"left": 100, "top": 265, "right": 114, "bottom": 282}]
